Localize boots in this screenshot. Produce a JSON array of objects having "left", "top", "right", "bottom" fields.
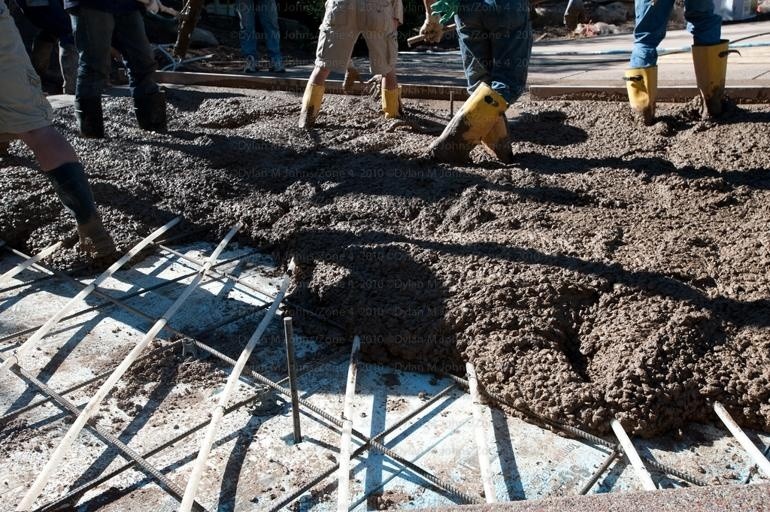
[
  {"left": 624, "top": 65, "right": 660, "bottom": 126},
  {"left": 132, "top": 89, "right": 169, "bottom": 135},
  {"left": 422, "top": 81, "right": 515, "bottom": 165},
  {"left": 690, "top": 39, "right": 735, "bottom": 121},
  {"left": 381, "top": 85, "right": 404, "bottom": 120},
  {"left": 76, "top": 96, "right": 104, "bottom": 137},
  {"left": 298, "top": 83, "right": 326, "bottom": 129}
]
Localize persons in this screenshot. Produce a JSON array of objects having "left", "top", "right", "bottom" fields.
[
  {"left": 0, "top": 0, "right": 115, "bottom": 261},
  {"left": 63, "top": 0, "right": 168, "bottom": 135},
  {"left": 298, "top": 0, "right": 403, "bottom": 129},
  {"left": 17, "top": 0, "right": 76, "bottom": 94},
  {"left": 624, "top": 0, "right": 731, "bottom": 123},
  {"left": 236, "top": 0, "right": 286, "bottom": 73},
  {"left": 420, "top": 0, "right": 533, "bottom": 165}
]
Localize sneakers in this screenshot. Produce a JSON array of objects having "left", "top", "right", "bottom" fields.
[
  {"left": 243, "top": 55, "right": 258, "bottom": 74},
  {"left": 76, "top": 227, "right": 116, "bottom": 260},
  {"left": 269, "top": 58, "right": 283, "bottom": 73}
]
